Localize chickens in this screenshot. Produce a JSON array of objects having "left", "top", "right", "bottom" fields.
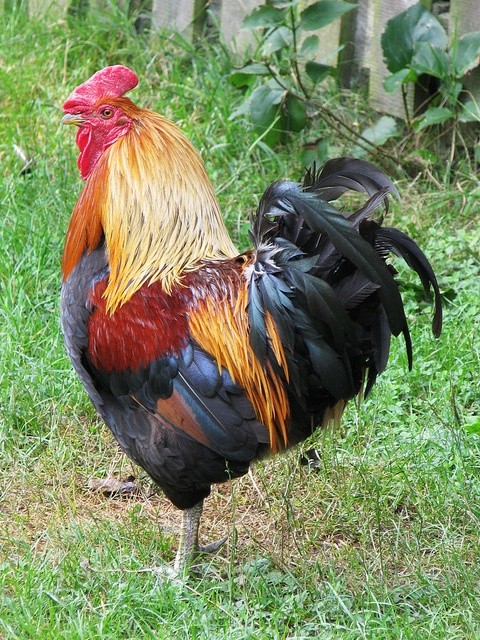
[{"left": 60, "top": 64, "right": 443, "bottom": 603}]
